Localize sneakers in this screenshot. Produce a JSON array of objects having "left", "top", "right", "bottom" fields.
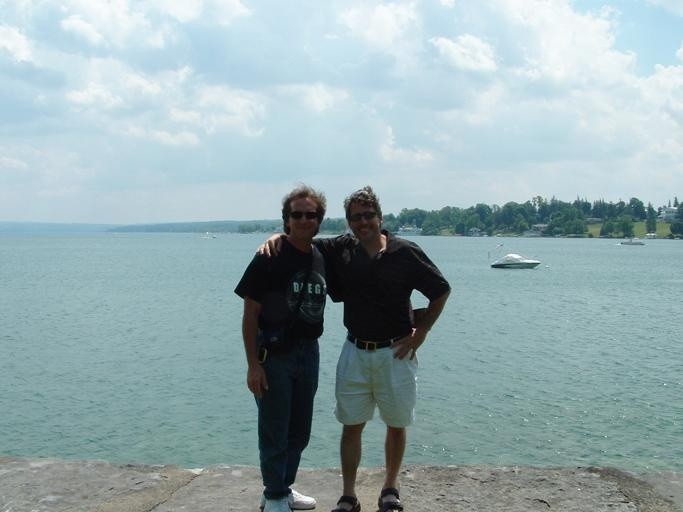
[
  {"left": 259, "top": 488, "right": 316, "bottom": 510},
  {"left": 262, "top": 495, "right": 292, "bottom": 512}
]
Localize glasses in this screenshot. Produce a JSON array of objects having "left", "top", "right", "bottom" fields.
[
  {"left": 350, "top": 211, "right": 376, "bottom": 221},
  {"left": 290, "top": 211, "right": 318, "bottom": 220}
]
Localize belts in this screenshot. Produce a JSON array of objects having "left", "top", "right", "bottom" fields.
[{"left": 347, "top": 330, "right": 413, "bottom": 353}]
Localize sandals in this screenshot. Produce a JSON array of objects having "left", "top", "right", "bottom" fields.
[
  {"left": 330, "top": 495, "right": 361, "bottom": 512},
  {"left": 378, "top": 487, "right": 403, "bottom": 512}
]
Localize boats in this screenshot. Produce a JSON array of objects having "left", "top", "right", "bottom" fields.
[
  {"left": 620, "top": 237, "right": 645, "bottom": 245},
  {"left": 490, "top": 254, "right": 541, "bottom": 269}
]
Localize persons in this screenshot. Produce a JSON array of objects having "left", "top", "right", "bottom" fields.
[
  {"left": 234, "top": 186, "right": 327, "bottom": 512},
  {"left": 259, "top": 186, "right": 451, "bottom": 512}
]
